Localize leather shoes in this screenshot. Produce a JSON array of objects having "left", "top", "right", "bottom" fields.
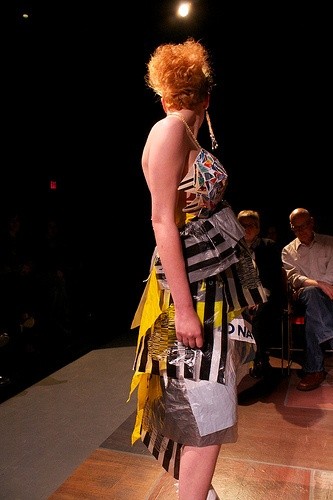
[
  {"left": 250, "top": 355, "right": 270, "bottom": 378},
  {"left": 298, "top": 371, "right": 326, "bottom": 391}
]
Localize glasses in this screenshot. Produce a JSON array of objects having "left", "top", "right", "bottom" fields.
[{"left": 290, "top": 221, "right": 310, "bottom": 231}]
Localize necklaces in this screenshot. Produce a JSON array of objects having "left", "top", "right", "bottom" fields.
[{"left": 167, "top": 113, "right": 202, "bottom": 148}]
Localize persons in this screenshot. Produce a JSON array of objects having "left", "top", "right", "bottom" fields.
[
  {"left": 7, "top": 214, "right": 25, "bottom": 241},
  {"left": 125, "top": 36, "right": 268, "bottom": 500},
  {"left": 235, "top": 208, "right": 333, "bottom": 390}
]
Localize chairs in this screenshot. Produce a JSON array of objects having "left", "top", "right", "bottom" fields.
[{"left": 261, "top": 312, "right": 333, "bottom": 376}]
